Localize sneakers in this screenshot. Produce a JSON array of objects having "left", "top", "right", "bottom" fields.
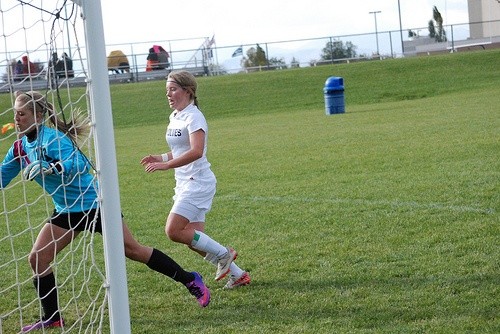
[
  {"left": 216, "top": 270, "right": 251, "bottom": 291},
  {"left": 215, "top": 247, "right": 238, "bottom": 281},
  {"left": 23, "top": 316, "right": 65, "bottom": 331},
  {"left": 185, "top": 272, "right": 211, "bottom": 307}
]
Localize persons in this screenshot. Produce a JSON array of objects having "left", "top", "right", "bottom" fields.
[
  {"left": 0, "top": 91, "right": 211, "bottom": 332},
  {"left": 14, "top": 90, "right": 22, "bottom": 97},
  {"left": 147, "top": 47, "right": 169, "bottom": 69},
  {"left": 140, "top": 71, "right": 250, "bottom": 290},
  {"left": 49, "top": 52, "right": 73, "bottom": 78},
  {"left": 13, "top": 56, "right": 36, "bottom": 80}
]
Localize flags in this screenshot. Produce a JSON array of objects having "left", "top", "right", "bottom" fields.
[{"left": 232, "top": 47, "right": 242, "bottom": 56}]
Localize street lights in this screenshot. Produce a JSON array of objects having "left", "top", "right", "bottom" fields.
[{"left": 369, "top": 11, "right": 381, "bottom": 60}]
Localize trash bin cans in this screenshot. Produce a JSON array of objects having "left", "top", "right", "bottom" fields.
[{"left": 323, "top": 76, "right": 345, "bottom": 115}]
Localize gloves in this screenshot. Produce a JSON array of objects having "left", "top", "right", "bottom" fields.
[{"left": 23, "top": 160, "right": 65, "bottom": 181}]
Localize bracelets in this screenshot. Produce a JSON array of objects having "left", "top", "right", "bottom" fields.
[{"left": 161, "top": 153, "right": 168, "bottom": 162}]
